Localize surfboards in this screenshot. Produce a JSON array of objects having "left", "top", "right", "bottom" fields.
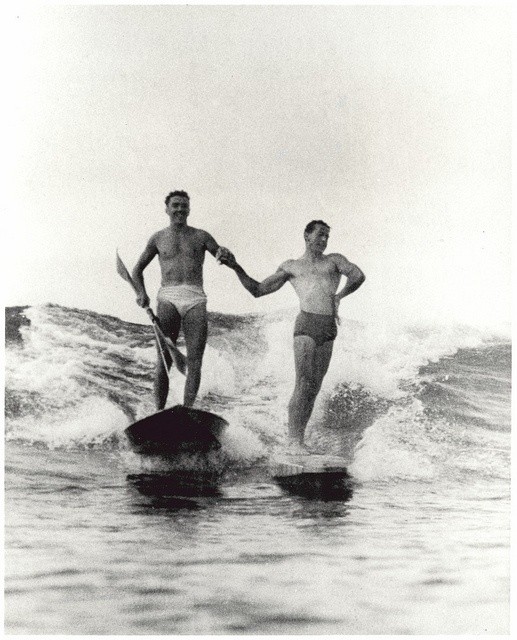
[{"left": 271, "top": 454, "right": 346, "bottom": 478}]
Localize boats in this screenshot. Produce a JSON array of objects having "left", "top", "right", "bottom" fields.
[{"left": 123, "top": 404, "right": 229, "bottom": 456}]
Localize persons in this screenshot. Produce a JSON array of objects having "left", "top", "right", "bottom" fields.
[
  {"left": 131, "top": 190, "right": 229, "bottom": 413},
  {"left": 214, "top": 219, "right": 365, "bottom": 456}
]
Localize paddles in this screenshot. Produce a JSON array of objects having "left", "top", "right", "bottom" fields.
[{"left": 116, "top": 247, "right": 188, "bottom": 376}]
